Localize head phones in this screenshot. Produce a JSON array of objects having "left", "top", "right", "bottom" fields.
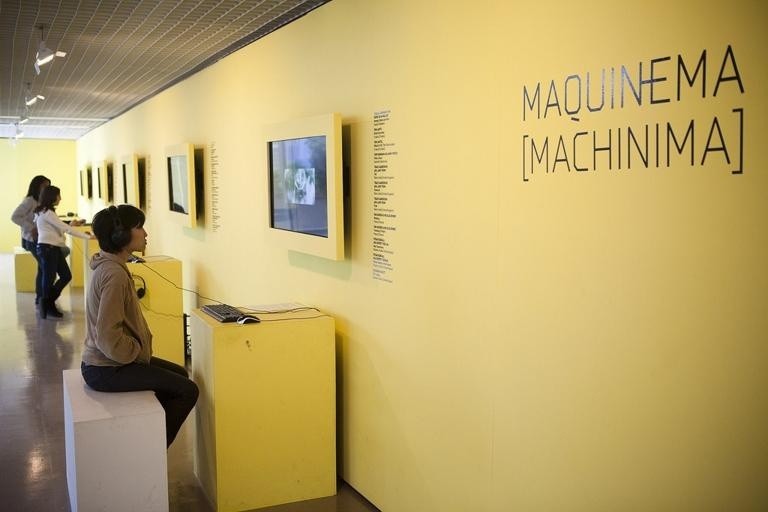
[
  {"left": 132, "top": 274, "right": 146, "bottom": 299},
  {"left": 108, "top": 205, "right": 131, "bottom": 248}
]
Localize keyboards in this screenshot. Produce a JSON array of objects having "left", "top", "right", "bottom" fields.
[
  {"left": 127, "top": 254, "right": 138, "bottom": 262},
  {"left": 200, "top": 303, "right": 246, "bottom": 323}
]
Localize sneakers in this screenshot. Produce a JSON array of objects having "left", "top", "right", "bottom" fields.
[{"left": 36, "top": 297, "right": 64, "bottom": 320}]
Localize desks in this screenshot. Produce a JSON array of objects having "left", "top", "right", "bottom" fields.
[
  {"left": 117, "top": 255, "right": 186, "bottom": 367},
  {"left": 81, "top": 233, "right": 145, "bottom": 313},
  {"left": 189, "top": 303, "right": 341, "bottom": 511},
  {"left": 62, "top": 224, "right": 97, "bottom": 290}
]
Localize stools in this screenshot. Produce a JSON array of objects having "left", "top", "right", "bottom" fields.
[
  {"left": 13, "top": 247, "right": 41, "bottom": 294},
  {"left": 54, "top": 367, "right": 175, "bottom": 512}
]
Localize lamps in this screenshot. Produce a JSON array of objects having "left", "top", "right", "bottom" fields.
[
  {"left": 21, "top": 81, "right": 46, "bottom": 109},
  {"left": 17, "top": 114, "right": 31, "bottom": 127},
  {"left": 27, "top": 23, "right": 68, "bottom": 75}
]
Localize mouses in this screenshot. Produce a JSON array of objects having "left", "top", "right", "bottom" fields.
[
  {"left": 237, "top": 314, "right": 261, "bottom": 324},
  {"left": 135, "top": 258, "right": 145, "bottom": 262}
]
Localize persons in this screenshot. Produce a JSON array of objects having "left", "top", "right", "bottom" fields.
[
  {"left": 79, "top": 204, "right": 199, "bottom": 451},
  {"left": 10, "top": 175, "right": 80, "bottom": 304},
  {"left": 34, "top": 186, "right": 93, "bottom": 319}
]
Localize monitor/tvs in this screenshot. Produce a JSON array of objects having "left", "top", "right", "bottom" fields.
[
  {"left": 97, "top": 167, "right": 113, "bottom": 203},
  {"left": 121, "top": 158, "right": 146, "bottom": 210},
  {"left": 262, "top": 111, "right": 345, "bottom": 262},
  {"left": 164, "top": 144, "right": 197, "bottom": 230},
  {"left": 80, "top": 169, "right": 92, "bottom": 199}
]
